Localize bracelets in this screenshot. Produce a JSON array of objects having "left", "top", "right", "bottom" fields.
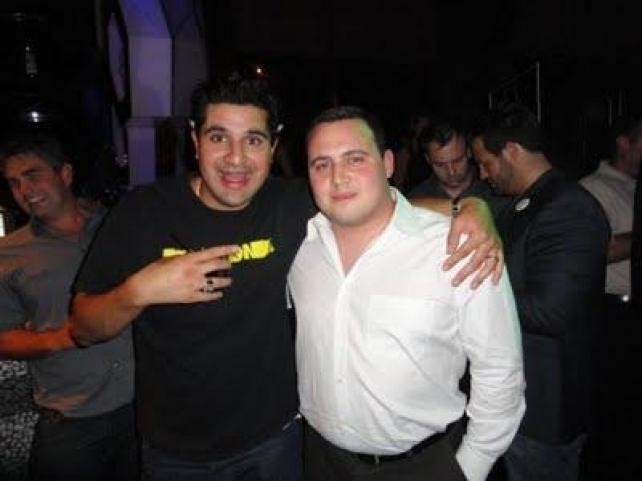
[{"left": 452, "top": 195, "right": 464, "bottom": 218}]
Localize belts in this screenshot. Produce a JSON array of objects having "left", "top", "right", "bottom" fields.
[{"left": 353, "top": 425, "right": 455, "bottom": 463}]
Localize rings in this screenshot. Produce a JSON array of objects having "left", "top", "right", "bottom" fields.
[
  {"left": 204, "top": 277, "right": 215, "bottom": 292},
  {"left": 485, "top": 255, "right": 499, "bottom": 261}
]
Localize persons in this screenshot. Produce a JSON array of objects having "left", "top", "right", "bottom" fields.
[
  {"left": 580, "top": 105, "right": 639, "bottom": 481},
  {"left": 285, "top": 100, "right": 528, "bottom": 480},
  {"left": 68, "top": 75, "right": 505, "bottom": 480},
  {"left": 409, "top": 116, "right": 487, "bottom": 202},
  {"left": 0, "top": 127, "right": 135, "bottom": 481},
  {"left": 471, "top": 104, "right": 612, "bottom": 480}
]
